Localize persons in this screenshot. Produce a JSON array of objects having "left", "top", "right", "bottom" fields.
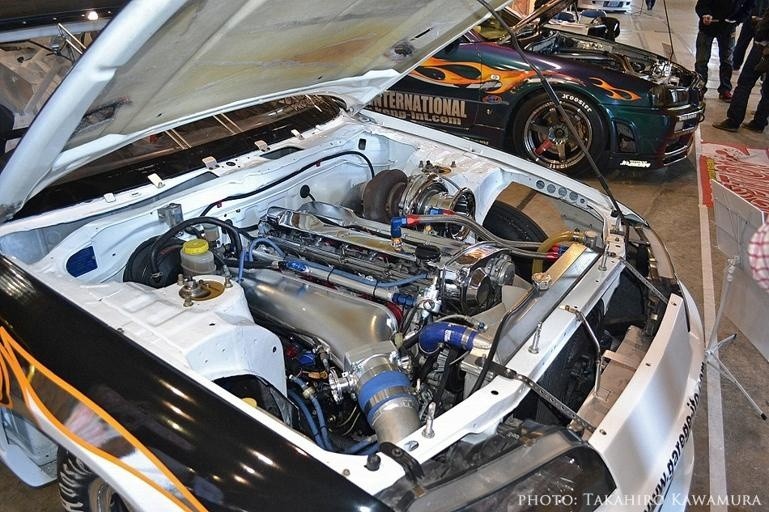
[
  {"left": 732, "top": 0, "right": 769, "bottom": 85},
  {"left": 641, "top": 1, "right": 657, "bottom": 17},
  {"left": 711, "top": 1, "right": 769, "bottom": 134},
  {"left": 693, "top": 1, "right": 750, "bottom": 105}
]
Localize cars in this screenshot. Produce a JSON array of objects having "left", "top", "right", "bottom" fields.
[
  {"left": 0, "top": 0, "right": 703, "bottom": 512},
  {"left": 362, "top": 1, "right": 707, "bottom": 177}
]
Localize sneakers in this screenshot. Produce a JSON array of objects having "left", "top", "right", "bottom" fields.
[
  {"left": 742, "top": 120, "right": 764, "bottom": 133},
  {"left": 712, "top": 117, "right": 739, "bottom": 132},
  {"left": 718, "top": 91, "right": 732, "bottom": 103}
]
